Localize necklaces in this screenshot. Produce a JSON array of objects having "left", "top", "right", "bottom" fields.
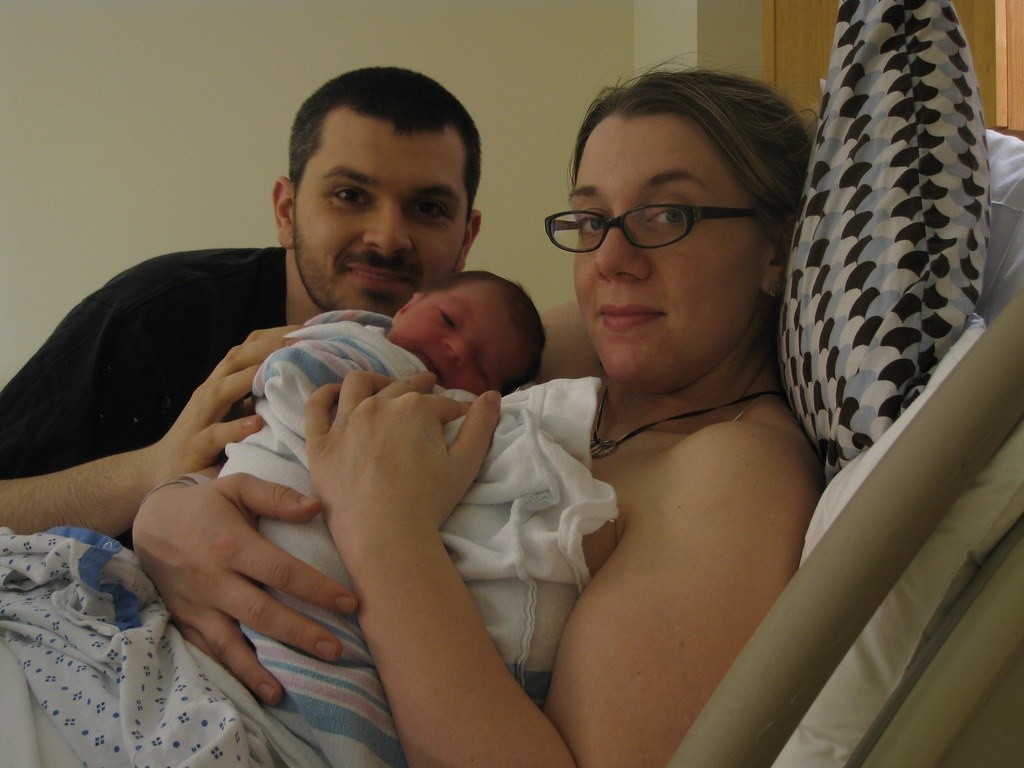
[{"left": 591, "top": 385, "right": 788, "bottom": 458}]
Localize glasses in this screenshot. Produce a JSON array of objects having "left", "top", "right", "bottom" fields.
[{"left": 544, "top": 203, "right": 762, "bottom": 253}]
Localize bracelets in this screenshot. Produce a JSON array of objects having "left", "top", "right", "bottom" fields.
[{"left": 138, "top": 472, "right": 209, "bottom": 508}]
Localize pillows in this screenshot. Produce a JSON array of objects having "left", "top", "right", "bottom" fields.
[{"left": 778, "top": 0, "right": 990, "bottom": 486}]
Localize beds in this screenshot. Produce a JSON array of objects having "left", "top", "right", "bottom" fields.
[{"left": 0, "top": 131, "right": 1024, "bottom": 768}]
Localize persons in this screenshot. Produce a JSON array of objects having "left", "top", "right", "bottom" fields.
[
  {"left": 220, "top": 270, "right": 545, "bottom": 768},
  {"left": 0, "top": 68, "right": 482, "bottom": 542},
  {"left": 0, "top": 73, "right": 821, "bottom": 767}
]
